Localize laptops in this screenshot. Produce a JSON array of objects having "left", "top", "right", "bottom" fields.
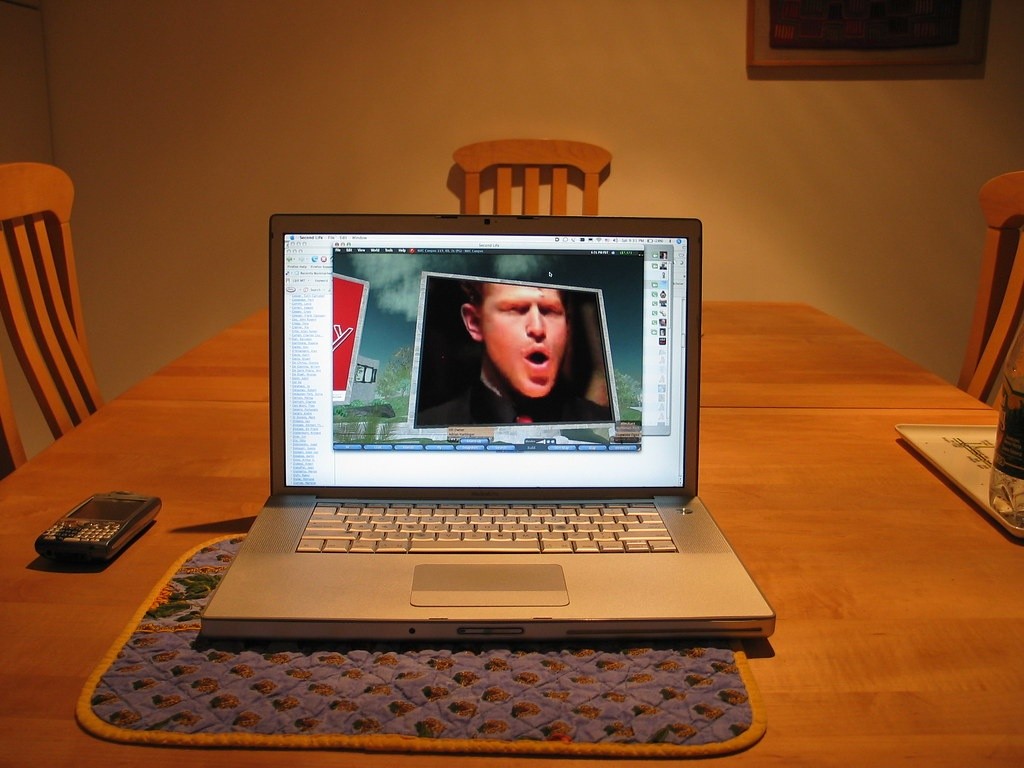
[{"left": 198, "top": 216, "right": 777, "bottom": 641}]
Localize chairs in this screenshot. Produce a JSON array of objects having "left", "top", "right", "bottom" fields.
[
  {"left": 0, "top": 162, "right": 103, "bottom": 481},
  {"left": 452, "top": 139, "right": 613, "bottom": 216},
  {"left": 956, "top": 171, "right": 1024, "bottom": 410}
]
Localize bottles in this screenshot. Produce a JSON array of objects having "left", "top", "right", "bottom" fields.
[{"left": 988, "top": 321, "right": 1024, "bottom": 530}]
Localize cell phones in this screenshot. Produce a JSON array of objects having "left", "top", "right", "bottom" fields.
[{"left": 35, "top": 492, "right": 163, "bottom": 560}]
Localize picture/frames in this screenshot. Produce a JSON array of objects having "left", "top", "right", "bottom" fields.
[{"left": 746, "top": 0, "right": 987, "bottom": 68}]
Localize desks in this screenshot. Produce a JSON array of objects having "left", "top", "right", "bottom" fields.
[
  {"left": 107, "top": 301, "right": 997, "bottom": 410},
  {"left": 0, "top": 409, "right": 1024, "bottom": 768}
]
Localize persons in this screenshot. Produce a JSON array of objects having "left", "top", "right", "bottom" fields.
[{"left": 414, "top": 278, "right": 613, "bottom": 429}]
